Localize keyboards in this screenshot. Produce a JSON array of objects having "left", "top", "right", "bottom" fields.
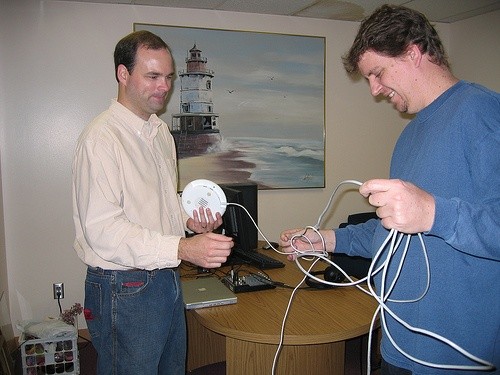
[{"left": 233, "top": 248, "right": 285, "bottom": 268}]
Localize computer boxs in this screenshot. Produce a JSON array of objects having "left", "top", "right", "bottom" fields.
[{"left": 219, "top": 184, "right": 258, "bottom": 249}]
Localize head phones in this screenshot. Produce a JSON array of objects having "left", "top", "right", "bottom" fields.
[{"left": 305, "top": 265, "right": 351, "bottom": 288}]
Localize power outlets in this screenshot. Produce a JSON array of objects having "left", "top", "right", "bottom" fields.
[{"left": 53, "top": 282, "right": 64, "bottom": 299}]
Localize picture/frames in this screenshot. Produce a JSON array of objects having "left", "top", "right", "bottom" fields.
[{"left": 133, "top": 22, "right": 326, "bottom": 192}]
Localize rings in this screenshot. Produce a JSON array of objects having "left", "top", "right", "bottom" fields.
[{"left": 202, "top": 226, "right": 208, "bottom": 229}]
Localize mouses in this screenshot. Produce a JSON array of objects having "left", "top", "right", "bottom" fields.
[{"left": 263, "top": 242, "right": 279, "bottom": 249}]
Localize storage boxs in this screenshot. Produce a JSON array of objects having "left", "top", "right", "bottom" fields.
[{"left": 18, "top": 313, "right": 81, "bottom": 375}]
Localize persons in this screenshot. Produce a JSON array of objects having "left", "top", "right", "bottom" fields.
[
  {"left": 72, "top": 30, "right": 236, "bottom": 374},
  {"left": 278, "top": 4, "right": 500, "bottom": 375}
]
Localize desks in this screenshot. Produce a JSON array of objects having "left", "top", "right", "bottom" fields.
[{"left": 177, "top": 238, "right": 384, "bottom": 375}]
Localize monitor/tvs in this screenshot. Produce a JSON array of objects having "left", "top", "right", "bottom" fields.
[{"left": 221, "top": 188, "right": 246, "bottom": 245}]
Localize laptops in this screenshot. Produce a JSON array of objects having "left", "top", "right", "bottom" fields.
[{"left": 182, "top": 277, "right": 237, "bottom": 310}]
